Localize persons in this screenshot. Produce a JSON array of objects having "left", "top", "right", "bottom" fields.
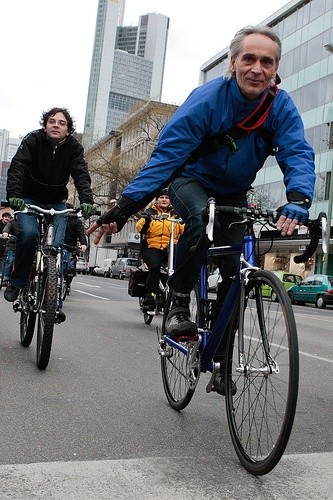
[
  {"left": 136, "top": 188, "right": 187, "bottom": 306},
  {"left": 3, "top": 107, "right": 96, "bottom": 322},
  {"left": 1, "top": 210, "right": 24, "bottom": 282},
  {"left": 84, "top": 26, "right": 317, "bottom": 395},
  {"left": 62, "top": 201, "right": 87, "bottom": 293}
]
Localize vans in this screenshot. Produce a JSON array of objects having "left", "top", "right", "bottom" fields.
[
  {"left": 248, "top": 271, "right": 303, "bottom": 302},
  {"left": 75, "top": 256, "right": 142, "bottom": 279}
]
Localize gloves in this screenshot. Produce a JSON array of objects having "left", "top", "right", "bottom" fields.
[
  {"left": 9, "top": 198, "right": 24, "bottom": 211},
  {"left": 79, "top": 202, "right": 97, "bottom": 220}
]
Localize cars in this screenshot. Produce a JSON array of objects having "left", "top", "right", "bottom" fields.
[
  {"left": 207, "top": 267, "right": 223, "bottom": 292},
  {"left": 286, "top": 274, "right": 333, "bottom": 309}
]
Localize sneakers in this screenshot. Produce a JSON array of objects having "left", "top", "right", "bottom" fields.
[
  {"left": 213, "top": 368, "right": 236, "bottom": 395},
  {"left": 165, "top": 308, "right": 197, "bottom": 335}
]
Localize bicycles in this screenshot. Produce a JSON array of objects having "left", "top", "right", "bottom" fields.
[
  {"left": 0, "top": 202, "right": 88, "bottom": 371},
  {"left": 138, "top": 213, "right": 183, "bottom": 325},
  {"left": 157, "top": 198, "right": 326, "bottom": 475}
]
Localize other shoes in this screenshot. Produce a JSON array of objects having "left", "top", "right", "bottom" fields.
[
  {"left": 4, "top": 284, "right": 19, "bottom": 301},
  {"left": 57, "top": 310, "right": 65, "bottom": 322},
  {"left": 2, "top": 281, "right": 8, "bottom": 286},
  {"left": 144, "top": 294, "right": 155, "bottom": 306}
]
